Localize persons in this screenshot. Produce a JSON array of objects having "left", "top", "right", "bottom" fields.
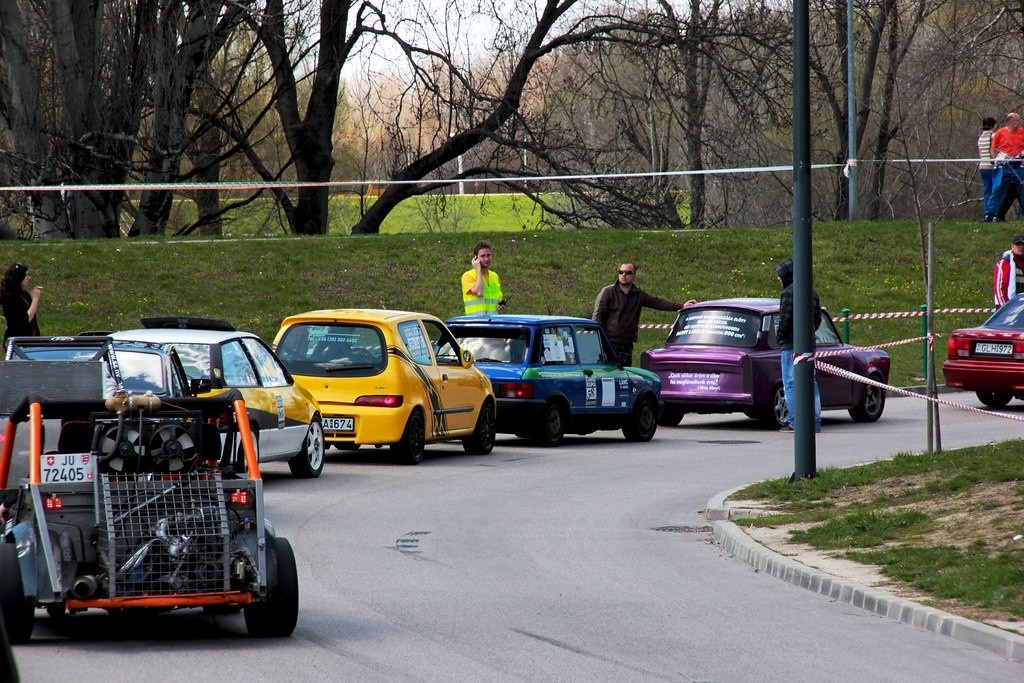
[
  {"left": 993, "top": 234, "right": 1024, "bottom": 312},
  {"left": 592, "top": 261, "right": 697, "bottom": 367},
  {"left": 984, "top": 114, "right": 1024, "bottom": 223},
  {"left": 978, "top": 118, "right": 997, "bottom": 214},
  {"left": 992, "top": 119, "right": 1024, "bottom": 223},
  {"left": 0, "top": 262, "right": 41, "bottom": 353},
  {"left": 773, "top": 258, "right": 822, "bottom": 434},
  {"left": 461, "top": 240, "right": 507, "bottom": 316}
]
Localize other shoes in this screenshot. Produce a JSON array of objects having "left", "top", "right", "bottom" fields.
[
  {"left": 779, "top": 426, "right": 795, "bottom": 432},
  {"left": 993, "top": 216, "right": 1007, "bottom": 222},
  {"left": 985, "top": 215, "right": 991, "bottom": 221}
]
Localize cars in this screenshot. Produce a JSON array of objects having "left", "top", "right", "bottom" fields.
[
  {"left": 69, "top": 317, "right": 325, "bottom": 478},
  {"left": 262, "top": 307, "right": 498, "bottom": 466},
  {"left": 427, "top": 313, "right": 662, "bottom": 444},
  {"left": 638, "top": 298, "right": 891, "bottom": 428},
  {"left": 940, "top": 292, "right": 1024, "bottom": 409}
]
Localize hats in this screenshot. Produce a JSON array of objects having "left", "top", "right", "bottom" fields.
[{"left": 1013, "top": 235, "right": 1024, "bottom": 244}]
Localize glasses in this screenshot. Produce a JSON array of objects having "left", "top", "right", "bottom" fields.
[
  {"left": 15, "top": 262, "right": 21, "bottom": 273},
  {"left": 619, "top": 270, "right": 635, "bottom": 275}
]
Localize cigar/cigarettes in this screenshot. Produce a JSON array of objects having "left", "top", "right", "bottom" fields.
[{"left": 38, "top": 286, "right": 44, "bottom": 289}]
